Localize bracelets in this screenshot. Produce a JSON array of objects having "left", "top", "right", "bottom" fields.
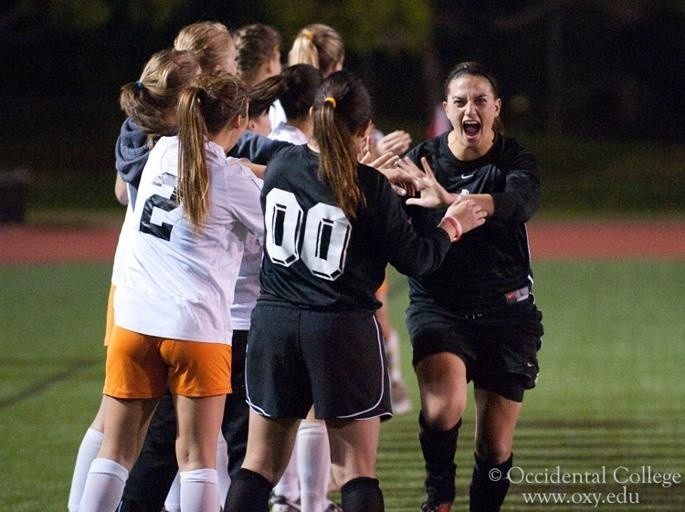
[{"left": 445, "top": 214, "right": 463, "bottom": 242}]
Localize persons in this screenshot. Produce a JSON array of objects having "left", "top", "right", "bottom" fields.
[
  {"left": 390, "top": 61, "right": 543, "bottom": 511},
  {"left": 67, "top": 22, "right": 413, "bottom": 512},
  {"left": 222, "top": 71, "right": 490, "bottom": 511}
]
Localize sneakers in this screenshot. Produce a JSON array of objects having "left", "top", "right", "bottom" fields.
[{"left": 420, "top": 497, "right": 454, "bottom": 512}]
